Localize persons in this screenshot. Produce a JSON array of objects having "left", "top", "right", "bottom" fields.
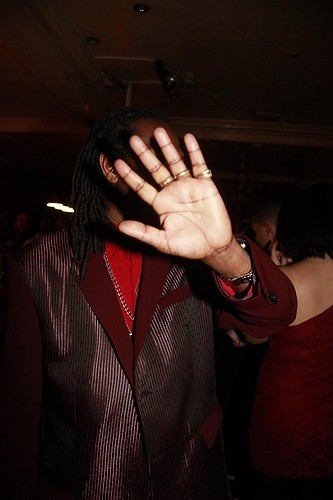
[
  {"left": 0, "top": 191, "right": 73, "bottom": 500},
  {"left": 18, "top": 106, "right": 298, "bottom": 500},
  {"left": 212, "top": 183, "right": 333, "bottom": 500}
]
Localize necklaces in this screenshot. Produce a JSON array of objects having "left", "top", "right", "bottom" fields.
[{"left": 103, "top": 251, "right": 135, "bottom": 320}]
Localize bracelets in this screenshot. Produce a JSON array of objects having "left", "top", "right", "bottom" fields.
[{"left": 218, "top": 241, "right": 255, "bottom": 285}]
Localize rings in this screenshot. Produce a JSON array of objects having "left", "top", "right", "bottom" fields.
[
  {"left": 158, "top": 176, "right": 174, "bottom": 188},
  {"left": 174, "top": 169, "right": 191, "bottom": 180},
  {"left": 198, "top": 169, "right": 211, "bottom": 177}
]
